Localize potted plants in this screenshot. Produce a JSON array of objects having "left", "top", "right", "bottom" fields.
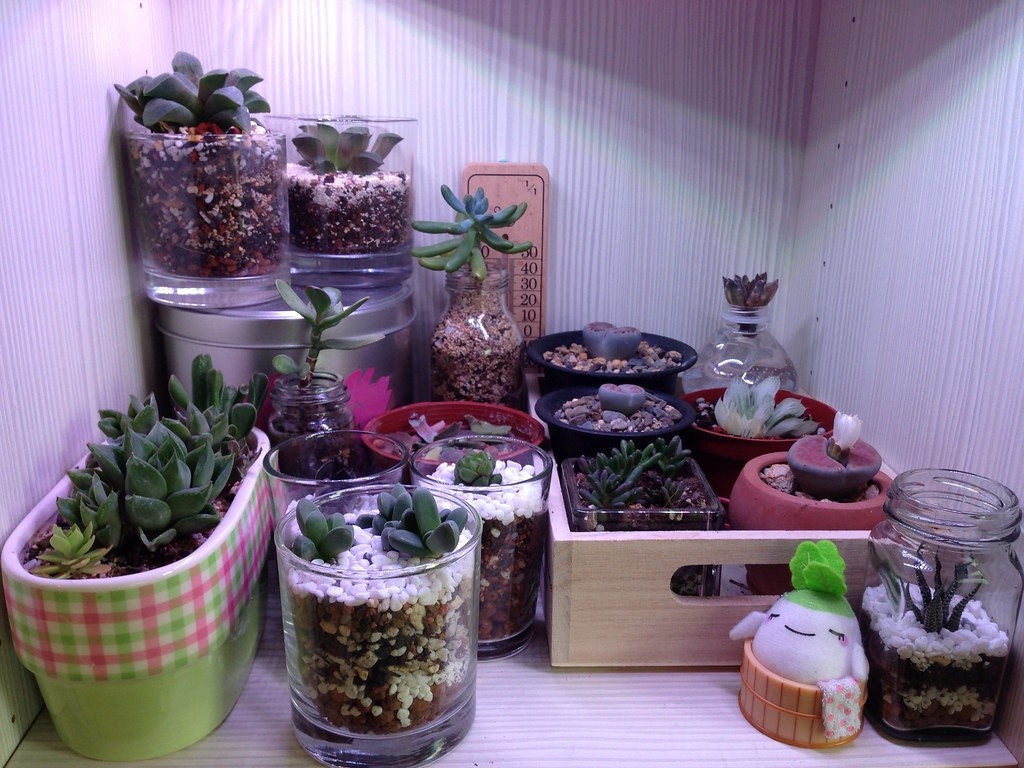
[
  {"left": 526, "top": 321, "right": 700, "bottom": 399},
  {"left": 857, "top": 469, "right": 1024, "bottom": 747},
  {"left": 363, "top": 402, "right": 547, "bottom": 483},
  {"left": 269, "top": 278, "right": 386, "bottom": 444},
  {"left": 728, "top": 410, "right": 896, "bottom": 595},
  {"left": 275, "top": 482, "right": 483, "bottom": 767},
  {"left": 561, "top": 437, "right": 727, "bottom": 599},
  {"left": 410, "top": 184, "right": 529, "bottom": 408},
  {"left": 684, "top": 374, "right": 839, "bottom": 481},
  {"left": 409, "top": 434, "right": 555, "bottom": 663},
  {"left": 113, "top": 52, "right": 290, "bottom": 307},
  {"left": 260, "top": 430, "right": 410, "bottom": 532},
  {"left": 682, "top": 272, "right": 800, "bottom": 392},
  {"left": 0, "top": 352, "right": 280, "bottom": 764},
  {"left": 534, "top": 382, "right": 695, "bottom": 459},
  {"left": 265, "top": 114, "right": 417, "bottom": 289}
]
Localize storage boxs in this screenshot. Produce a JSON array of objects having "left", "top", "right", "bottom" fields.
[{"left": 542, "top": 445, "right": 899, "bottom": 669}]
